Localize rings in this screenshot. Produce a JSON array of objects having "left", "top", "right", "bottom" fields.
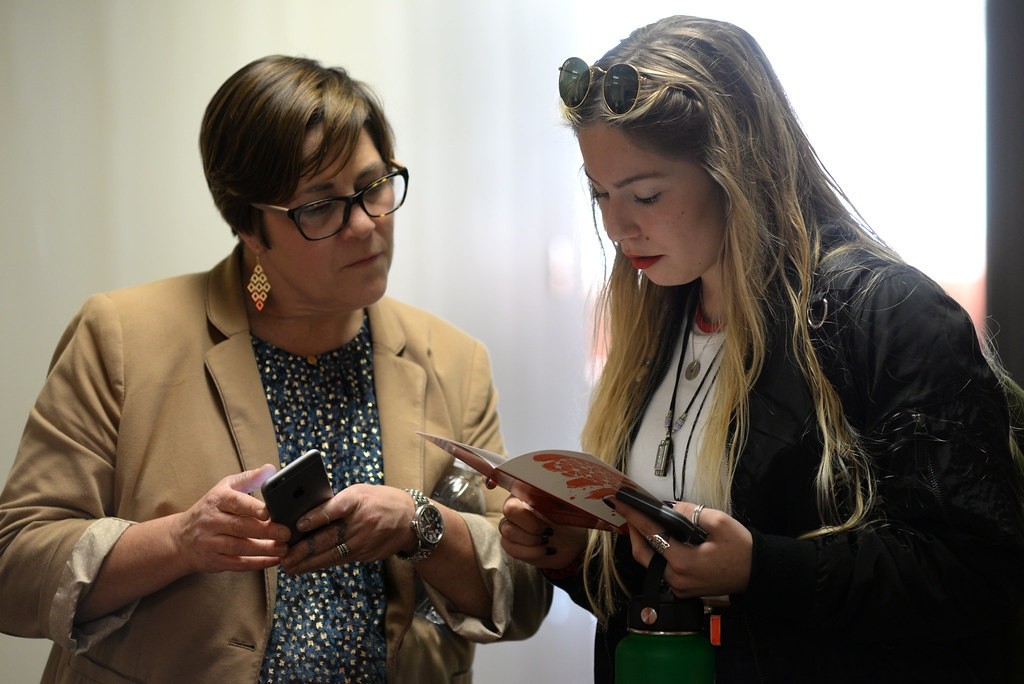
[
  {"left": 499, "top": 517, "right": 509, "bottom": 535},
  {"left": 337, "top": 542, "right": 350, "bottom": 558},
  {"left": 646, "top": 529, "right": 672, "bottom": 554},
  {"left": 692, "top": 502, "right": 705, "bottom": 527}
]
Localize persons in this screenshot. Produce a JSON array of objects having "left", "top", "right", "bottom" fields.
[
  {"left": 2, "top": 52, "right": 505, "bottom": 684},
  {"left": 498, "top": 15, "right": 1024, "bottom": 683}
]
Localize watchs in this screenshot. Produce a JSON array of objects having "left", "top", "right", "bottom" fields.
[{"left": 392, "top": 488, "right": 444, "bottom": 560}]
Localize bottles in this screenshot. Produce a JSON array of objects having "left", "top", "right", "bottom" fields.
[{"left": 613, "top": 598, "right": 716, "bottom": 684}]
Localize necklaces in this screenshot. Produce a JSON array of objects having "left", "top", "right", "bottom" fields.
[{"left": 653, "top": 282, "right": 748, "bottom": 503}]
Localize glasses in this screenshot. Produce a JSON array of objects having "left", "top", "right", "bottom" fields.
[
  {"left": 558, "top": 57, "right": 688, "bottom": 115},
  {"left": 227, "top": 160, "right": 409, "bottom": 241}
]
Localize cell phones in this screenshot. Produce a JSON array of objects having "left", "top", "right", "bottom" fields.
[
  {"left": 261, "top": 449, "right": 336, "bottom": 549},
  {"left": 617, "top": 486, "right": 708, "bottom": 547}
]
